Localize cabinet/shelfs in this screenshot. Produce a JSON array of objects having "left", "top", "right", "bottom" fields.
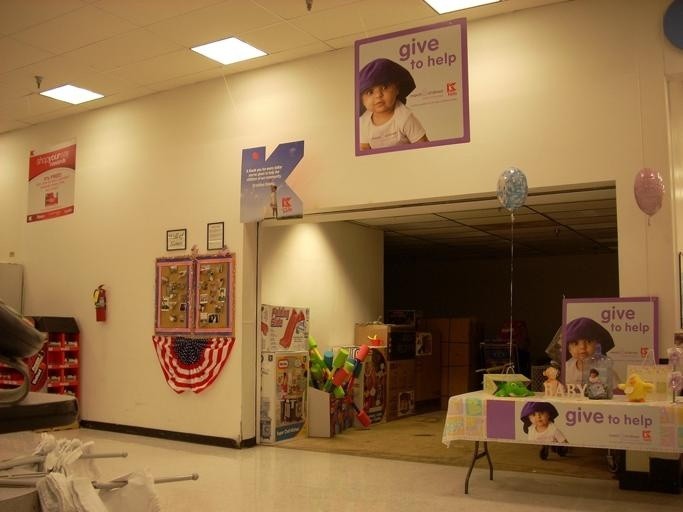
[
  {"left": 417, "top": 315, "right": 483, "bottom": 411},
  {"left": 0, "top": 315, "right": 81, "bottom": 405}
]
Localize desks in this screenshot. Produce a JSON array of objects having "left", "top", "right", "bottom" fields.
[
  {"left": 483, "top": 341, "right": 525, "bottom": 377},
  {"left": 442, "top": 390, "right": 683, "bottom": 493}
]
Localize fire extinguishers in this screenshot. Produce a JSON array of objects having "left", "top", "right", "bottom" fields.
[{"left": 93, "top": 285, "right": 106, "bottom": 321}]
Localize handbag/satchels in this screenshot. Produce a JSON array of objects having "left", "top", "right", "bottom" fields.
[
  {"left": 627, "top": 349, "right": 672, "bottom": 401},
  {"left": 483, "top": 364, "right": 531, "bottom": 395}
]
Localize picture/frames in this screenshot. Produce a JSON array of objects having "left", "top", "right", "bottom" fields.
[
  {"left": 207, "top": 222, "right": 224, "bottom": 250},
  {"left": 167, "top": 230, "right": 187, "bottom": 252}
]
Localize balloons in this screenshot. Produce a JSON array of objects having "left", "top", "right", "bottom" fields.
[
  {"left": 634, "top": 168, "right": 665, "bottom": 216},
  {"left": 497, "top": 166, "right": 528, "bottom": 212}
]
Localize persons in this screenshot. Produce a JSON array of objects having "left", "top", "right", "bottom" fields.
[
  {"left": 270, "top": 180, "right": 277, "bottom": 218},
  {"left": 567, "top": 318, "right": 621, "bottom": 385},
  {"left": 520, "top": 402, "right": 568, "bottom": 443},
  {"left": 588, "top": 368, "right": 601, "bottom": 383},
  {"left": 359, "top": 58, "right": 430, "bottom": 151}
]
